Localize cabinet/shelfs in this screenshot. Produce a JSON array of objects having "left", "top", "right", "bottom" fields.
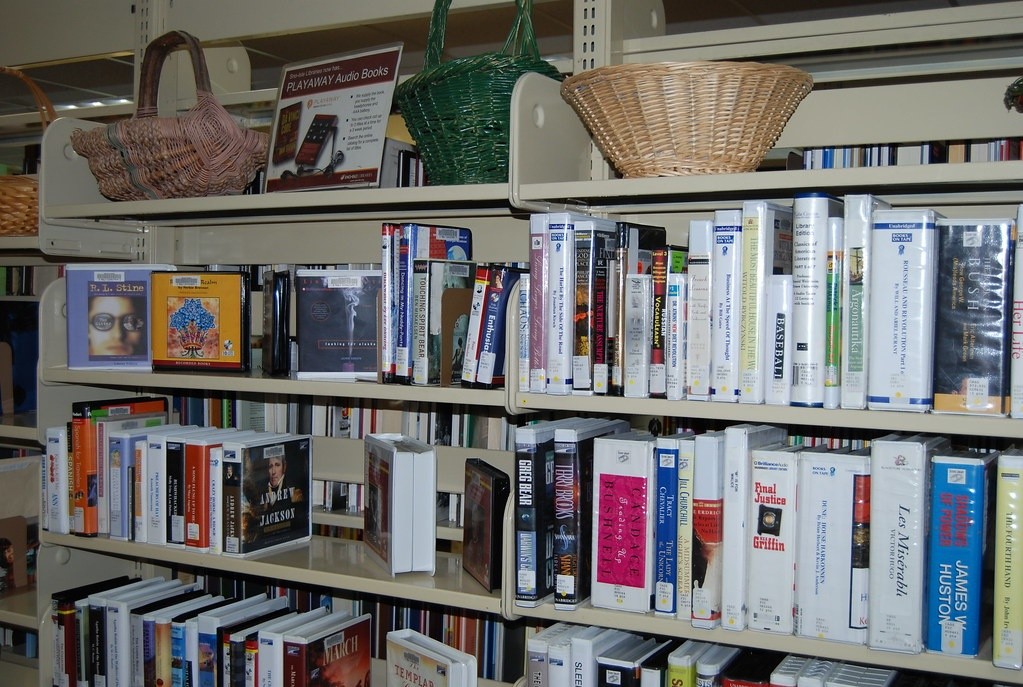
[{"left": 0, "top": 0, "right": 1023, "bottom": 687}]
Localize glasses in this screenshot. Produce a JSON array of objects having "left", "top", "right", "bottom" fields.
[{"left": 89, "top": 313, "right": 146, "bottom": 332}]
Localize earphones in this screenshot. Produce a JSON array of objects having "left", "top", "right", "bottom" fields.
[
  {"left": 281, "top": 170, "right": 298, "bottom": 181},
  {"left": 297, "top": 167, "right": 315, "bottom": 175}
]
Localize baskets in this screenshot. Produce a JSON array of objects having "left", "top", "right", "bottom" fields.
[
  {"left": 560, "top": 62, "right": 814, "bottom": 180},
  {"left": 71, "top": 30, "right": 271, "bottom": 202},
  {"left": 0, "top": 67, "right": 58, "bottom": 237},
  {"left": 392, "top": 0, "right": 569, "bottom": 186}
]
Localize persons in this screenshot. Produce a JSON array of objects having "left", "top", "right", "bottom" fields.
[
  {"left": 261, "top": 455, "right": 293, "bottom": 509},
  {"left": 0, "top": 537, "right": 20, "bottom": 600},
  {"left": 87, "top": 296, "right": 149, "bottom": 356},
  {"left": 221, "top": 463, "right": 240, "bottom": 487}
]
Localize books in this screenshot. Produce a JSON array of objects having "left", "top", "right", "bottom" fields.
[
  {"left": 802, "top": 138, "right": 1023, "bottom": 171},
  {"left": 40, "top": 191, "right": 1023, "bottom": 687}
]
